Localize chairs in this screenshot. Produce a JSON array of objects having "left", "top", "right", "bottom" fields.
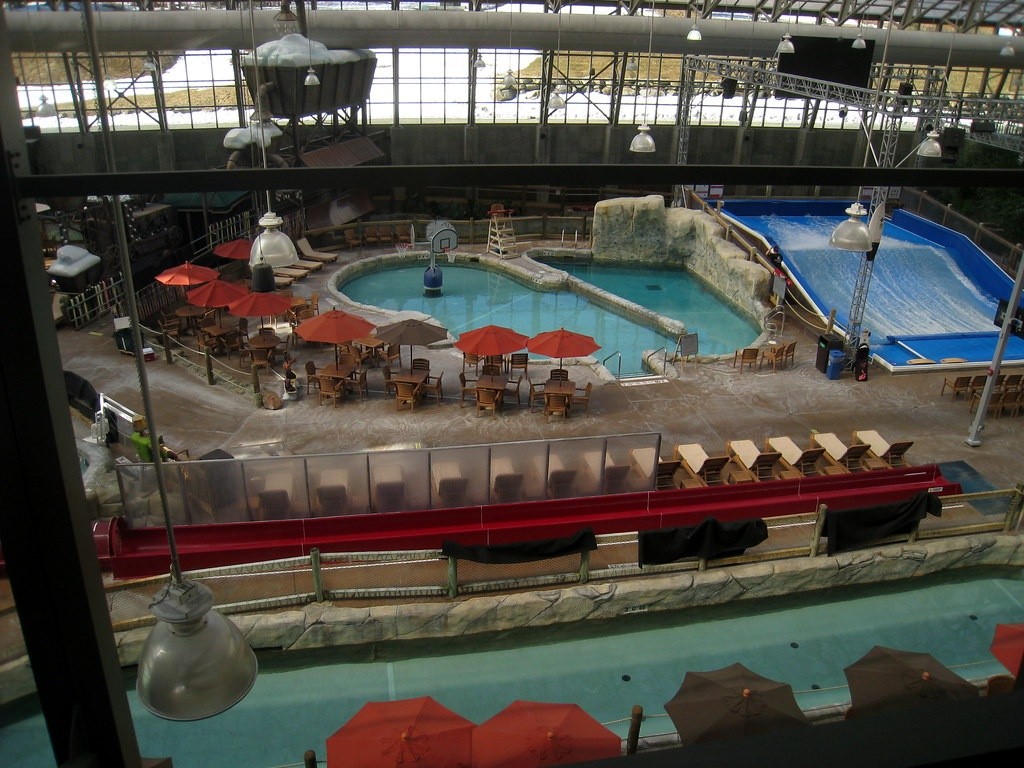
[
  {"left": 158, "top": 427, "right": 916, "bottom": 526},
  {"left": 155, "top": 290, "right": 594, "bottom": 426},
  {"left": 938, "top": 373, "right": 1024, "bottom": 421},
  {"left": 248, "top": 237, "right": 339, "bottom": 290},
  {"left": 727, "top": 339, "right": 800, "bottom": 376},
  {"left": 484, "top": 203, "right": 523, "bottom": 261}
]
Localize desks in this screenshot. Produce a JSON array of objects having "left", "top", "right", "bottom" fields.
[
  {"left": 318, "top": 363, "right": 357, "bottom": 400},
  {"left": 391, "top": 368, "right": 432, "bottom": 402},
  {"left": 353, "top": 334, "right": 392, "bottom": 369},
  {"left": 247, "top": 334, "right": 282, "bottom": 364},
  {"left": 542, "top": 379, "right": 576, "bottom": 418},
  {"left": 474, "top": 374, "right": 509, "bottom": 412},
  {"left": 175, "top": 305, "right": 205, "bottom": 337},
  {"left": 200, "top": 323, "right": 239, "bottom": 356}
]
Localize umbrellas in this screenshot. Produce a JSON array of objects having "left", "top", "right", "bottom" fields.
[
  {"left": 293, "top": 306, "right": 602, "bottom": 387},
  {"left": 324, "top": 695, "right": 622, "bottom": 768},
  {"left": 989, "top": 622, "right": 1024, "bottom": 685},
  {"left": 664, "top": 662, "right": 811, "bottom": 768},
  {"left": 844, "top": 646, "right": 982, "bottom": 756},
  {"left": 154, "top": 261, "right": 293, "bottom": 341},
  {"left": 213, "top": 238, "right": 255, "bottom": 283}
]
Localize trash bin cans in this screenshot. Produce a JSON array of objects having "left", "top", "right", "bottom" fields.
[
  {"left": 816, "top": 334, "right": 844, "bottom": 373},
  {"left": 199, "top": 449, "right": 238, "bottom": 508},
  {"left": 112, "top": 316, "right": 144, "bottom": 356},
  {"left": 826, "top": 350, "right": 846, "bottom": 380}
]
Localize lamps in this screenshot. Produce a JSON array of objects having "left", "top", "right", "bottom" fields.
[
  {"left": 73, "top": 0, "right": 259, "bottom": 726},
  {"left": 244, "top": 0, "right": 301, "bottom": 274},
  {"left": 16, "top": 0, "right": 1024, "bottom": 161},
  {"left": 826, "top": 0, "right": 898, "bottom": 255}
]
